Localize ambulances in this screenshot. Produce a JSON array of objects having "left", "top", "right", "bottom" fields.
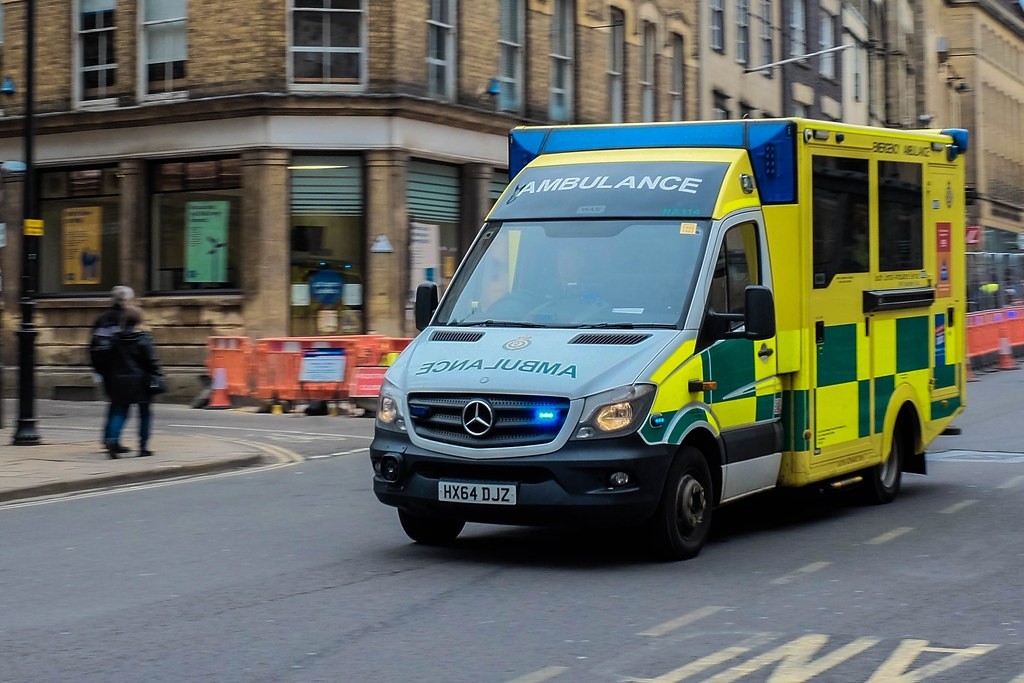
[{"left": 370, "top": 117, "right": 968, "bottom": 560}]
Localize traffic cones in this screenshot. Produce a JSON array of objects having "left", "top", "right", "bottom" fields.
[
  {"left": 993, "top": 324, "right": 1020, "bottom": 368},
  {"left": 202, "top": 349, "right": 232, "bottom": 409}
]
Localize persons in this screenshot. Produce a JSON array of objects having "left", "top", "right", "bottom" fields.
[{"left": 89, "top": 285, "right": 164, "bottom": 457}]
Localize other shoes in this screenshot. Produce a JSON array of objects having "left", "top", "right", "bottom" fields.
[
  {"left": 139, "top": 449, "right": 151, "bottom": 455},
  {"left": 106, "top": 442, "right": 128, "bottom": 450},
  {"left": 110, "top": 441, "right": 118, "bottom": 457}
]
